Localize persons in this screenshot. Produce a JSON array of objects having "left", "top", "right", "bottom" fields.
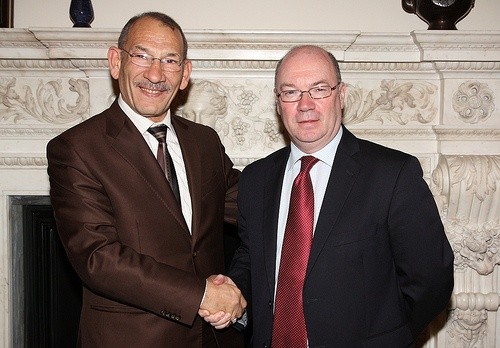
[
  {"left": 46, "top": 12, "right": 248, "bottom": 348},
  {"left": 197, "top": 45, "right": 454, "bottom": 348}
]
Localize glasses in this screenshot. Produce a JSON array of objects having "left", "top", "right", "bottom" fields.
[
  {"left": 275, "top": 83, "right": 341, "bottom": 103},
  {"left": 120, "top": 47, "right": 185, "bottom": 71}
]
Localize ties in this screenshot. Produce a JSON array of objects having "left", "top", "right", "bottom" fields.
[
  {"left": 271, "top": 156, "right": 319, "bottom": 348},
  {"left": 147, "top": 124, "right": 182, "bottom": 214}
]
